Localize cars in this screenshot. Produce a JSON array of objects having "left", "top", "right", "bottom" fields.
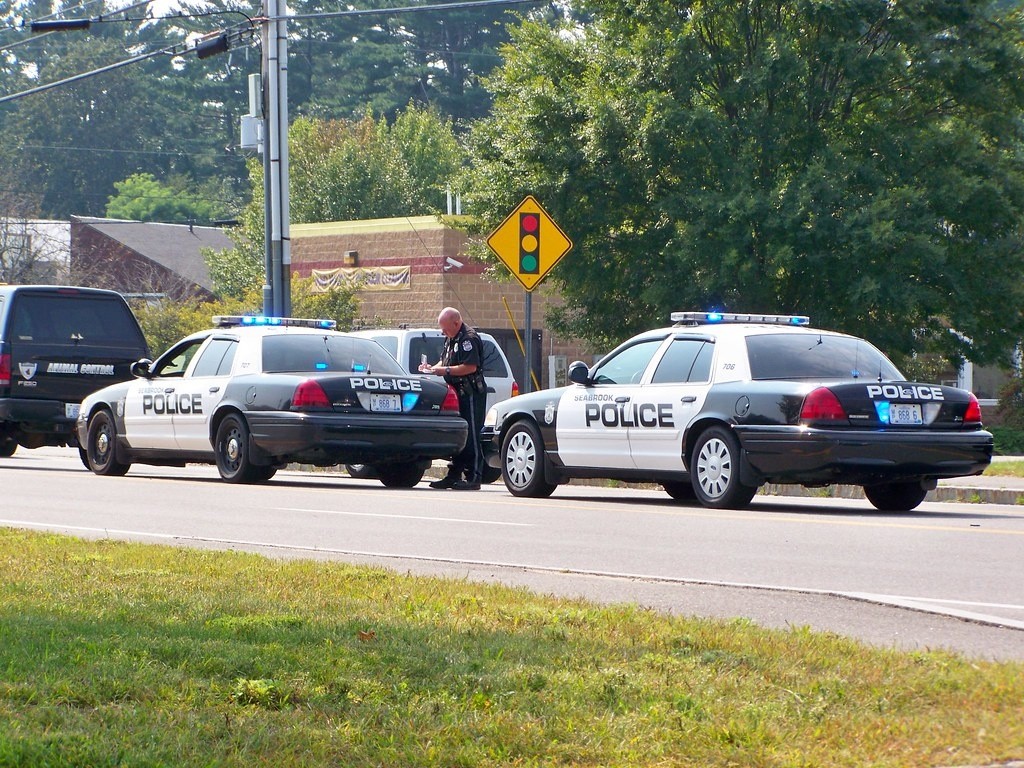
[
  {"left": 77, "top": 316, "right": 469, "bottom": 489},
  {"left": 477, "top": 312, "right": 994, "bottom": 511}
]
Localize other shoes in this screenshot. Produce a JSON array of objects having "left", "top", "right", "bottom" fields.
[
  {"left": 453, "top": 476, "right": 481, "bottom": 490},
  {"left": 430, "top": 473, "right": 459, "bottom": 488}
]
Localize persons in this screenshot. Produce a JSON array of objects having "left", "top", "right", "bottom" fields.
[{"left": 418, "top": 306, "right": 488, "bottom": 491}]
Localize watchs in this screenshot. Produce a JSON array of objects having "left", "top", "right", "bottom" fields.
[{"left": 445, "top": 365, "right": 451, "bottom": 376}]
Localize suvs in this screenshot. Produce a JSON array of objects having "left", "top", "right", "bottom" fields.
[
  {"left": 348, "top": 329, "right": 519, "bottom": 485},
  {"left": 0, "top": 285, "right": 151, "bottom": 472}
]
